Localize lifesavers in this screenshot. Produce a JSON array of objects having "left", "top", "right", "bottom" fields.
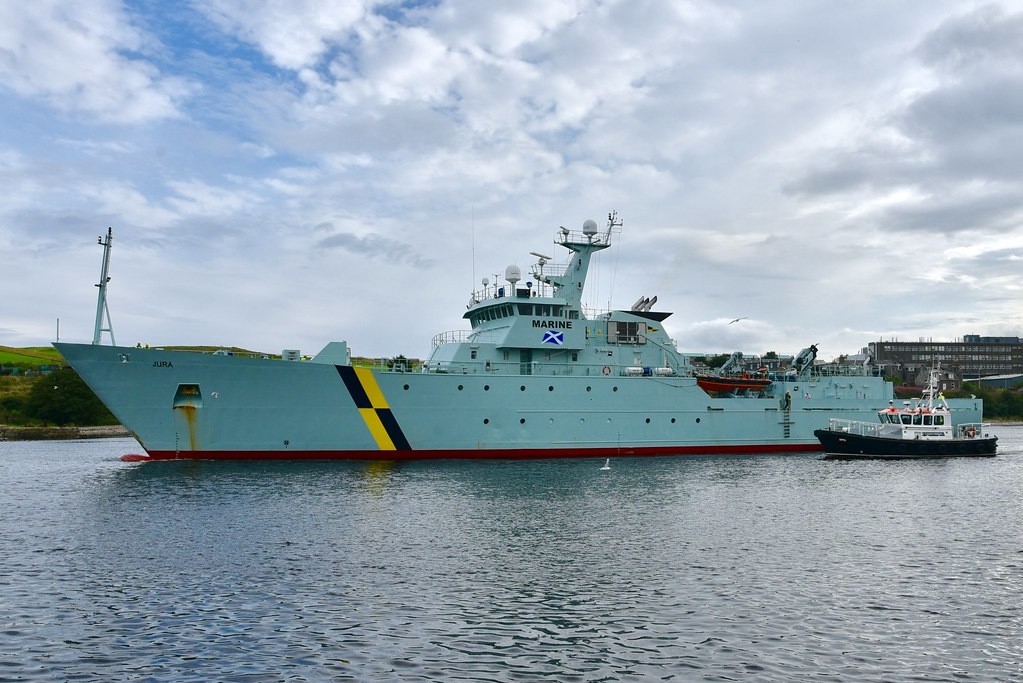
[
  {"left": 602, "top": 366, "right": 611, "bottom": 375},
  {"left": 977, "top": 443, "right": 985, "bottom": 452},
  {"left": 919, "top": 445, "right": 928, "bottom": 453},
  {"left": 958, "top": 444, "right": 967, "bottom": 453},
  {"left": 897, "top": 443, "right": 908, "bottom": 453},
  {"left": 938, "top": 445, "right": 948, "bottom": 454}
]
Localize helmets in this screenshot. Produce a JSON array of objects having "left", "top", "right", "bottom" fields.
[{"left": 939, "top": 392, "right": 943, "bottom": 394}]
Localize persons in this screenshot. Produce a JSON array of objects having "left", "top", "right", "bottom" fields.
[
  {"left": 134, "top": 342, "right": 142, "bottom": 348},
  {"left": 301, "top": 355, "right": 307, "bottom": 360},
  {"left": 142, "top": 343, "right": 150, "bottom": 349},
  {"left": 783, "top": 390, "right": 791, "bottom": 408},
  {"left": 754, "top": 371, "right": 758, "bottom": 378},
  {"left": 227, "top": 348, "right": 234, "bottom": 356},
  {"left": 758, "top": 389, "right": 764, "bottom": 398},
  {"left": 763, "top": 371, "right": 767, "bottom": 376},
  {"left": 805, "top": 392, "right": 811, "bottom": 399}
]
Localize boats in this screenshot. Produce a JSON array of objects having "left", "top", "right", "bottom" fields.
[
  {"left": 768, "top": 374, "right": 799, "bottom": 383},
  {"left": 697, "top": 373, "right": 772, "bottom": 392},
  {"left": 53, "top": 206, "right": 984, "bottom": 464},
  {"left": 814, "top": 349, "right": 999, "bottom": 459}
]
